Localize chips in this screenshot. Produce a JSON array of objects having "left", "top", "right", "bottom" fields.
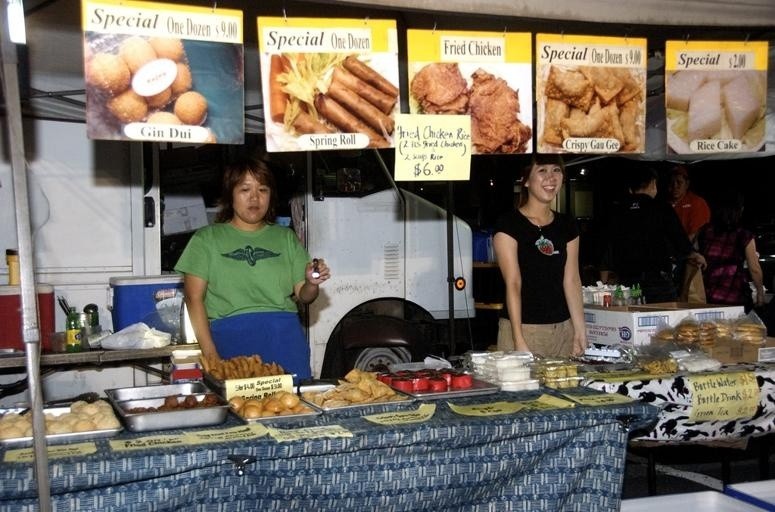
[{"left": 303, "top": 368, "right": 403, "bottom": 409}]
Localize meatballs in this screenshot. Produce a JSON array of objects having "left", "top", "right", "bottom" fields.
[{"left": 126, "top": 395, "right": 218, "bottom": 414}]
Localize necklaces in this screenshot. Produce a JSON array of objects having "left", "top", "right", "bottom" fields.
[{"left": 521, "top": 205, "right": 553, "bottom": 228}]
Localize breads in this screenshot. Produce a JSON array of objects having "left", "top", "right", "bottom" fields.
[
  {"left": 543, "top": 67, "right": 645, "bottom": 151},
  {"left": 0, "top": 399, "right": 119, "bottom": 440},
  {"left": 86, "top": 36, "right": 207, "bottom": 128},
  {"left": 230, "top": 390, "right": 311, "bottom": 419}
]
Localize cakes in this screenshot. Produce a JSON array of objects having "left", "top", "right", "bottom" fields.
[{"left": 377, "top": 368, "right": 473, "bottom": 394}]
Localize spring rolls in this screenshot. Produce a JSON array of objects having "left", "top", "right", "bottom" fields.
[
  {"left": 196, "top": 352, "right": 284, "bottom": 380},
  {"left": 292, "top": 57, "right": 392, "bottom": 146}
]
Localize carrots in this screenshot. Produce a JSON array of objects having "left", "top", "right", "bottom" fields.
[{"left": 269, "top": 55, "right": 289, "bottom": 122}]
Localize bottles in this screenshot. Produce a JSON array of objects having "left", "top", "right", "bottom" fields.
[
  {"left": 5, "top": 247, "right": 21, "bottom": 286},
  {"left": 65, "top": 306, "right": 81, "bottom": 352},
  {"left": 601, "top": 284, "right": 646, "bottom": 307}
]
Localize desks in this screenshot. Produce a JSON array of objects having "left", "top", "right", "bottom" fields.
[
  {"left": 463, "top": 336, "right": 775, "bottom": 495},
  {"left": 1, "top": 367, "right": 661, "bottom": 512}
]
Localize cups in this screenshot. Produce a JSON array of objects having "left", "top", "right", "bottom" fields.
[
  {"left": 49, "top": 332, "right": 67, "bottom": 353},
  {"left": 81, "top": 325, "right": 103, "bottom": 350}
]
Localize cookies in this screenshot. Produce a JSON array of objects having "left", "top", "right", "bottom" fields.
[{"left": 656, "top": 322, "right": 766, "bottom": 348}]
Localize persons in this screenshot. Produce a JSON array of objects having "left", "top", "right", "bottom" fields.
[
  {"left": 612, "top": 160, "right": 708, "bottom": 303},
  {"left": 173, "top": 156, "right": 331, "bottom": 384},
  {"left": 665, "top": 163, "right": 712, "bottom": 242},
  {"left": 492, "top": 154, "right": 590, "bottom": 358},
  {"left": 698, "top": 189, "right": 764, "bottom": 307}
]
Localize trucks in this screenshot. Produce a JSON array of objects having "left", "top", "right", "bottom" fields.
[{"left": 0, "top": 0, "right": 480, "bottom": 386}]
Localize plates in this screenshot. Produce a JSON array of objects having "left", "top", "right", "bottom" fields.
[{"left": 666, "top": 119, "right": 765, "bottom": 155}]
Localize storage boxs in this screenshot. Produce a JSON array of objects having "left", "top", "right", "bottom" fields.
[
  {"left": 105, "top": 275, "right": 187, "bottom": 345},
  {"left": 576, "top": 299, "right": 744, "bottom": 348},
  {"left": 0, "top": 283, "right": 56, "bottom": 351}
]
